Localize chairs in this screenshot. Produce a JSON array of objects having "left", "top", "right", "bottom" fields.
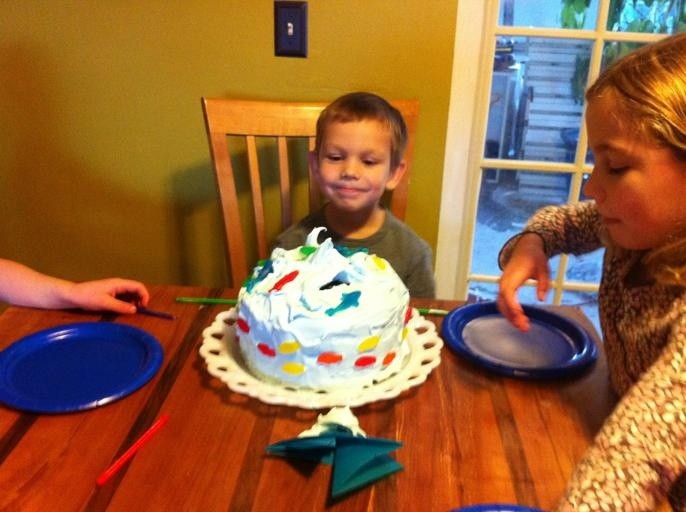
[{"left": 201, "top": 97, "right": 421, "bottom": 287}]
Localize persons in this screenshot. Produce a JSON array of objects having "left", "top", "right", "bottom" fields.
[
  {"left": 492, "top": 35, "right": 684, "bottom": 512},
  {"left": 0, "top": 257, "right": 151, "bottom": 316},
  {"left": 267, "top": 90, "right": 434, "bottom": 300}
]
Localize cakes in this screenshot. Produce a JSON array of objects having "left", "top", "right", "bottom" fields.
[{"left": 233, "top": 225, "right": 411, "bottom": 393}]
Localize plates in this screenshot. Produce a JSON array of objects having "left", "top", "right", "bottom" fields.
[
  {"left": 0, "top": 321, "right": 164, "bottom": 416},
  {"left": 441, "top": 303, "right": 599, "bottom": 385}
]
